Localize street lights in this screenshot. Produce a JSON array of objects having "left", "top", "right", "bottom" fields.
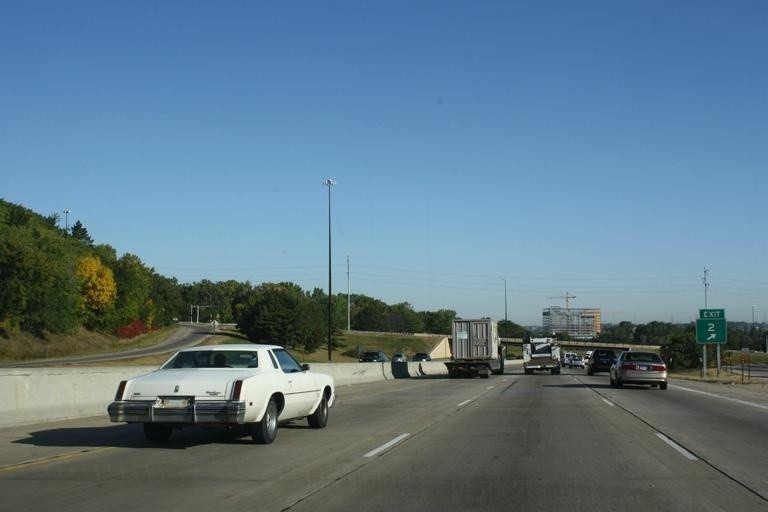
[
  {"left": 346, "top": 255, "right": 351, "bottom": 330},
  {"left": 62, "top": 209, "right": 72, "bottom": 240},
  {"left": 321, "top": 177, "right": 336, "bottom": 361},
  {"left": 500, "top": 278, "right": 508, "bottom": 357}
]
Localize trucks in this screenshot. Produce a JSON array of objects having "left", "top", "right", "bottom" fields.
[{"left": 444, "top": 317, "right": 506, "bottom": 377}]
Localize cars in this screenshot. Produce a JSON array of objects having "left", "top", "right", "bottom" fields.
[
  {"left": 107, "top": 344, "right": 336, "bottom": 444},
  {"left": 560, "top": 348, "right": 668, "bottom": 390},
  {"left": 411, "top": 352, "right": 431, "bottom": 361},
  {"left": 358, "top": 350, "right": 389, "bottom": 361},
  {"left": 391, "top": 352, "right": 407, "bottom": 362}
]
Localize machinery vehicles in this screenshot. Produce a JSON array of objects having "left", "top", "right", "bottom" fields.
[{"left": 522, "top": 336, "right": 562, "bottom": 375}]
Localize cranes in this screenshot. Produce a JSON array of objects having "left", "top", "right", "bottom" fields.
[{"left": 546, "top": 292, "right": 576, "bottom": 313}]
